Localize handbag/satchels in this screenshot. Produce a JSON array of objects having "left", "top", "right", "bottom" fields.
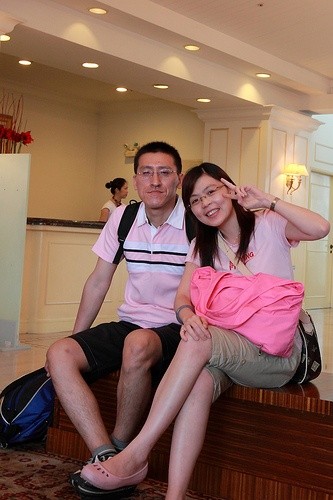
[
  {"left": 291, "top": 311, "right": 322, "bottom": 385},
  {"left": 190, "top": 265, "right": 304, "bottom": 358}
]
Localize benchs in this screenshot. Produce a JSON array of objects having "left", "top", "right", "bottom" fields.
[{"left": 44, "top": 372, "right": 333, "bottom": 500}]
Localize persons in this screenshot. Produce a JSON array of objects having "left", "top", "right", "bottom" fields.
[
  {"left": 100, "top": 178, "right": 128, "bottom": 222},
  {"left": 45, "top": 138, "right": 190, "bottom": 494},
  {"left": 80, "top": 159, "right": 330, "bottom": 500}
]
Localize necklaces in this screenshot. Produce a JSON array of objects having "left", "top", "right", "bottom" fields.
[{"left": 222, "top": 233, "right": 240, "bottom": 256}]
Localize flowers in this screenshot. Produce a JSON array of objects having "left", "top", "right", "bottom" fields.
[{"left": 0, "top": 88, "right": 34, "bottom": 353}]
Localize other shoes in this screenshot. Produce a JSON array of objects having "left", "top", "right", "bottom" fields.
[
  {"left": 69, "top": 449, "right": 135, "bottom": 496},
  {"left": 80, "top": 460, "right": 150, "bottom": 490}
]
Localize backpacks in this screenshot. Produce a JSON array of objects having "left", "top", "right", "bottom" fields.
[{"left": 0, "top": 366, "right": 54, "bottom": 449}]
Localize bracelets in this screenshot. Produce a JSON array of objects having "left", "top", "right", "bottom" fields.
[
  {"left": 270, "top": 197, "right": 281, "bottom": 212},
  {"left": 176, "top": 305, "right": 193, "bottom": 325}
]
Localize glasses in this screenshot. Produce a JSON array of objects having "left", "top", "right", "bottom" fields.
[
  {"left": 137, "top": 167, "right": 179, "bottom": 177},
  {"left": 188, "top": 184, "right": 226, "bottom": 210}
]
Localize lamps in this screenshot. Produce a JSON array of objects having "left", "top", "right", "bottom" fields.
[{"left": 280, "top": 164, "right": 309, "bottom": 196}]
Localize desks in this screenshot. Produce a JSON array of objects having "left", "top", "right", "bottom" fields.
[{"left": 19, "top": 217, "right": 130, "bottom": 334}]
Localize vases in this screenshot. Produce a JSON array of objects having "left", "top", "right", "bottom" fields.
[{"left": 0, "top": 153, "right": 34, "bottom": 353}]
